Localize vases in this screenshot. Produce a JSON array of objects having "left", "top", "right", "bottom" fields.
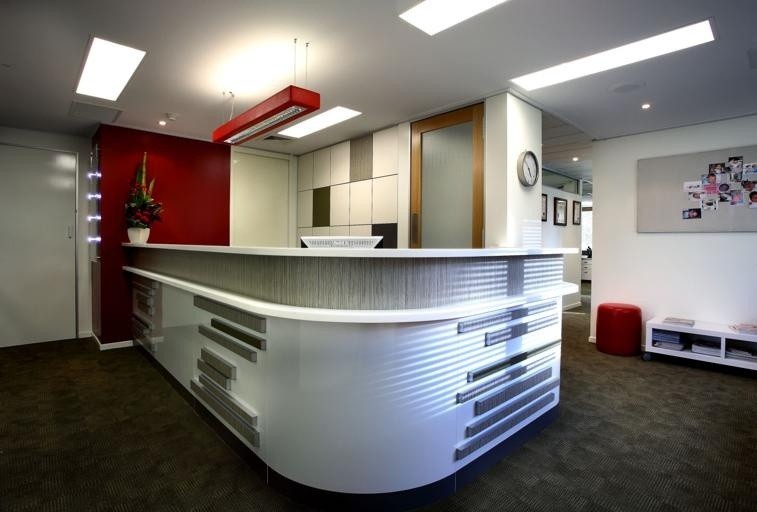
[{"left": 127, "top": 227, "right": 151, "bottom": 244}]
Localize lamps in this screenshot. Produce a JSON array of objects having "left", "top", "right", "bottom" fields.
[{"left": 213, "top": 38, "right": 320, "bottom": 146}]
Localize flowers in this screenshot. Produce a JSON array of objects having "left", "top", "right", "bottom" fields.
[{"left": 124, "top": 152, "right": 164, "bottom": 228}]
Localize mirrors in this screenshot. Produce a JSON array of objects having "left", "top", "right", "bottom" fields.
[{"left": 88, "top": 144, "right": 102, "bottom": 263}]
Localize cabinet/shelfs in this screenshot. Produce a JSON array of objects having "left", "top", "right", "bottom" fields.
[
  {"left": 582, "top": 258, "right": 592, "bottom": 280},
  {"left": 643, "top": 317, "right": 757, "bottom": 371}
]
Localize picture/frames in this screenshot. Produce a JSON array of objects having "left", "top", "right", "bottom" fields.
[{"left": 542, "top": 194, "right": 581, "bottom": 225}]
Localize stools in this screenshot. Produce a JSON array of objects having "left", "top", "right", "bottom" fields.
[{"left": 596, "top": 303, "right": 641, "bottom": 356}]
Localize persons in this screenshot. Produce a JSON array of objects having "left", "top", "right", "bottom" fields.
[{"left": 680, "top": 155, "right": 757, "bottom": 219}]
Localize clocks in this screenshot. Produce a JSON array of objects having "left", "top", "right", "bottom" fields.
[{"left": 517, "top": 151, "right": 539, "bottom": 187}]
